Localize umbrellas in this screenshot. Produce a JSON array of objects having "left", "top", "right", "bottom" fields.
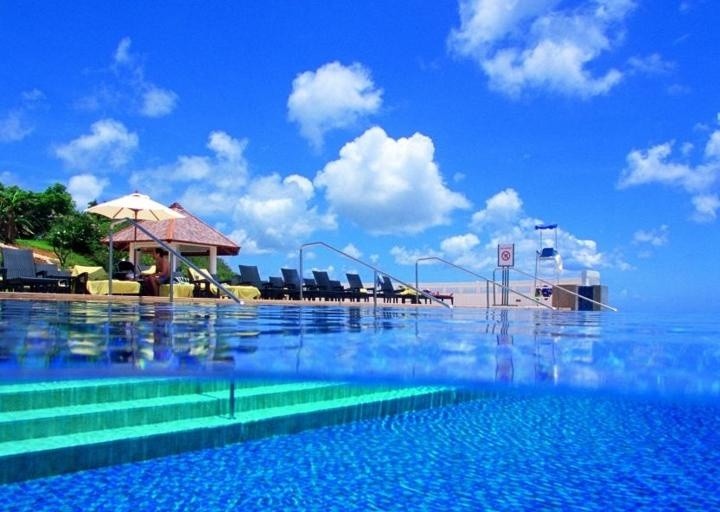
[{"left": 86, "top": 191, "right": 186, "bottom": 279}]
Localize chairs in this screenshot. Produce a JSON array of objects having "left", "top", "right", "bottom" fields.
[{"left": 0, "top": 247, "right": 453, "bottom": 303}]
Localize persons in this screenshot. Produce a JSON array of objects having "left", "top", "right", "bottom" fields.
[{"left": 145, "top": 246, "right": 170, "bottom": 295}]
